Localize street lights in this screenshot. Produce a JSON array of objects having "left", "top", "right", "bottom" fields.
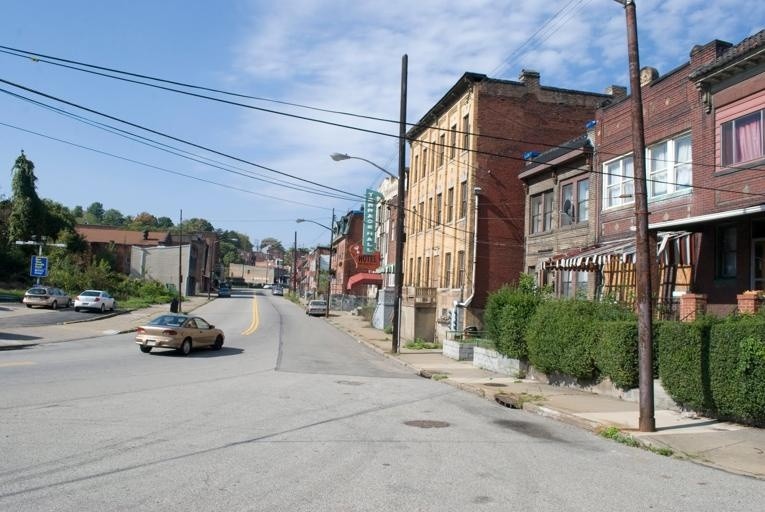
[
  {"left": 13, "top": 239, "right": 68, "bottom": 284},
  {"left": 327, "top": 151, "right": 406, "bottom": 354},
  {"left": 294, "top": 219, "right": 334, "bottom": 318},
  {"left": 207, "top": 237, "right": 240, "bottom": 301}
]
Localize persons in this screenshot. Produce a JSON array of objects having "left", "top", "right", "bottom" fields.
[{"left": 169, "top": 295, "right": 179, "bottom": 313}]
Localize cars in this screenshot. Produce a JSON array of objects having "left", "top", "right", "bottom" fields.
[
  {"left": 262, "top": 284, "right": 289, "bottom": 297},
  {"left": 303, "top": 299, "right": 328, "bottom": 315},
  {"left": 219, "top": 282, "right": 230, "bottom": 289},
  {"left": 72, "top": 289, "right": 117, "bottom": 313},
  {"left": 21, "top": 284, "right": 73, "bottom": 310},
  {"left": 133, "top": 313, "right": 228, "bottom": 357},
  {"left": 217, "top": 287, "right": 231, "bottom": 298}
]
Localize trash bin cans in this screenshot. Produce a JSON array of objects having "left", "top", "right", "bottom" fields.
[{"left": 170, "top": 297, "right": 178, "bottom": 313}]
